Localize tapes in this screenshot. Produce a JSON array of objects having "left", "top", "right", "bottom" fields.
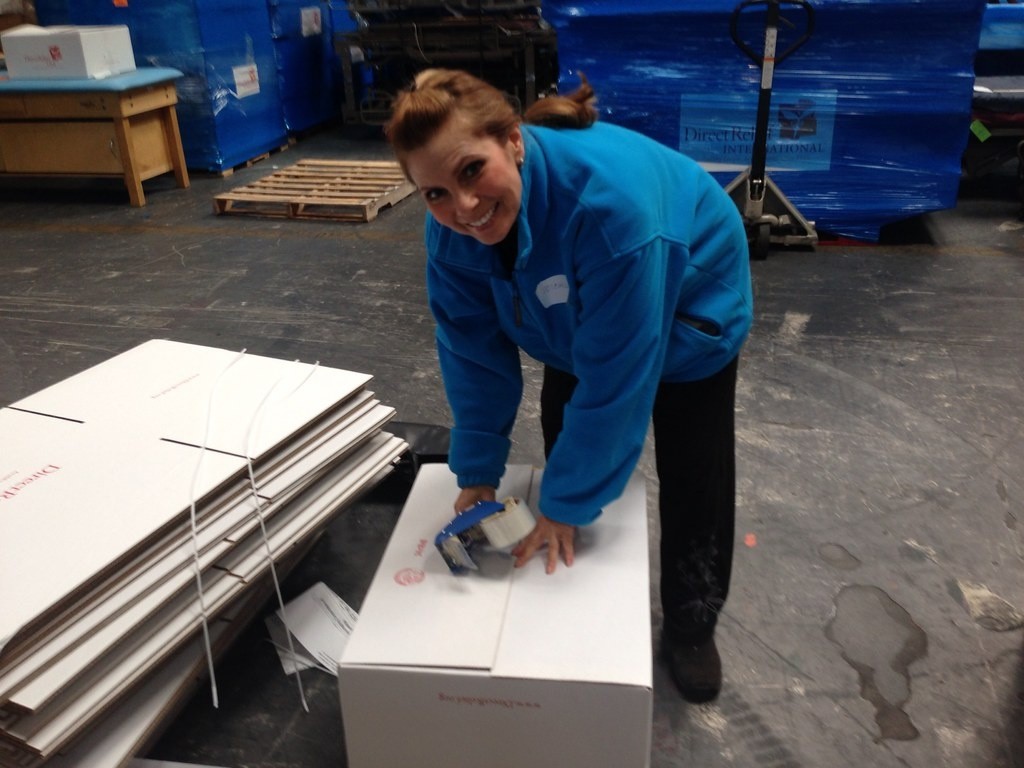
[{"left": 479, "top": 497, "right": 537, "bottom": 549}]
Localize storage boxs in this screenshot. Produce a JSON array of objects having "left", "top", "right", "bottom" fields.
[
  {"left": 338, "top": 464, "right": 654, "bottom": 768},
  {"left": 0, "top": 23, "right": 136, "bottom": 80}
]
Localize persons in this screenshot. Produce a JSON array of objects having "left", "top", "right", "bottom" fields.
[{"left": 383, "top": 69, "right": 754, "bottom": 704}]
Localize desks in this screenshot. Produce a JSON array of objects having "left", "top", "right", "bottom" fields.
[{"left": 0, "top": 66, "right": 191, "bottom": 207}]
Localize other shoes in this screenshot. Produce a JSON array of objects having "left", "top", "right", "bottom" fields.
[{"left": 662, "top": 629, "right": 721, "bottom": 703}]
